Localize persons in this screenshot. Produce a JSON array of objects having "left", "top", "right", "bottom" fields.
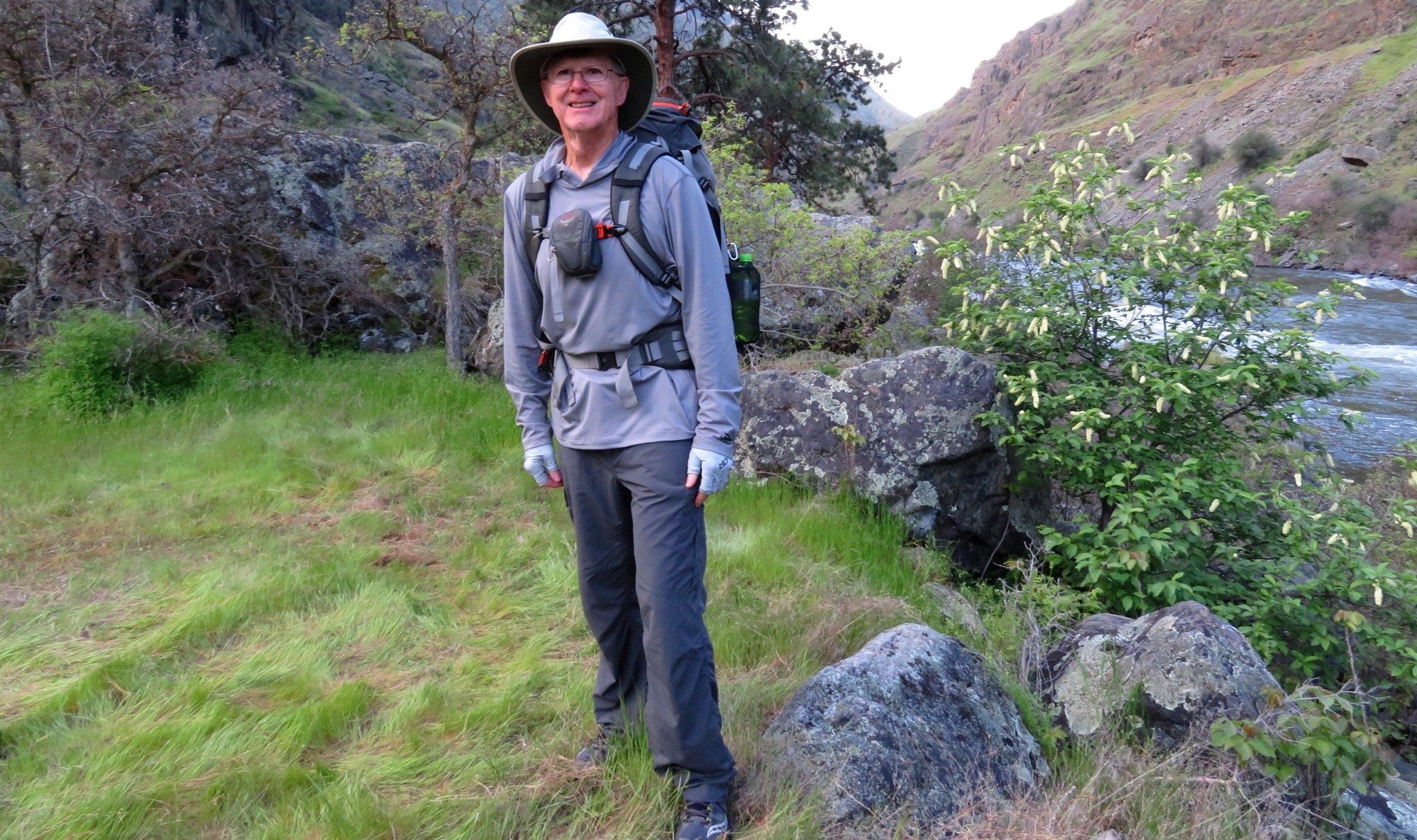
[{"left": 498, "top": 15, "right": 743, "bottom": 840}]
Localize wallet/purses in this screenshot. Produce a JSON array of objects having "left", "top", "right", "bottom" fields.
[{"left": 549, "top": 207, "right": 603, "bottom": 280}]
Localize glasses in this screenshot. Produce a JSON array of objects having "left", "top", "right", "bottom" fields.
[{"left": 543, "top": 62, "right": 622, "bottom": 84}]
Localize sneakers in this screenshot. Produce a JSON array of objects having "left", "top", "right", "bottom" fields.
[
  {"left": 674, "top": 797, "right": 730, "bottom": 839},
  {"left": 574, "top": 724, "right": 634, "bottom": 782}
]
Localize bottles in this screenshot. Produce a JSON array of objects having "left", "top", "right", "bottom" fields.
[{"left": 732, "top": 254, "right": 760, "bottom": 343}]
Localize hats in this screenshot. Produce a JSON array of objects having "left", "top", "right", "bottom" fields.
[{"left": 508, "top": 11, "right": 657, "bottom": 134}]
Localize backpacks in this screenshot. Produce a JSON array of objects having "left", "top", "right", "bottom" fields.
[{"left": 520, "top": 94, "right": 738, "bottom": 382}]
